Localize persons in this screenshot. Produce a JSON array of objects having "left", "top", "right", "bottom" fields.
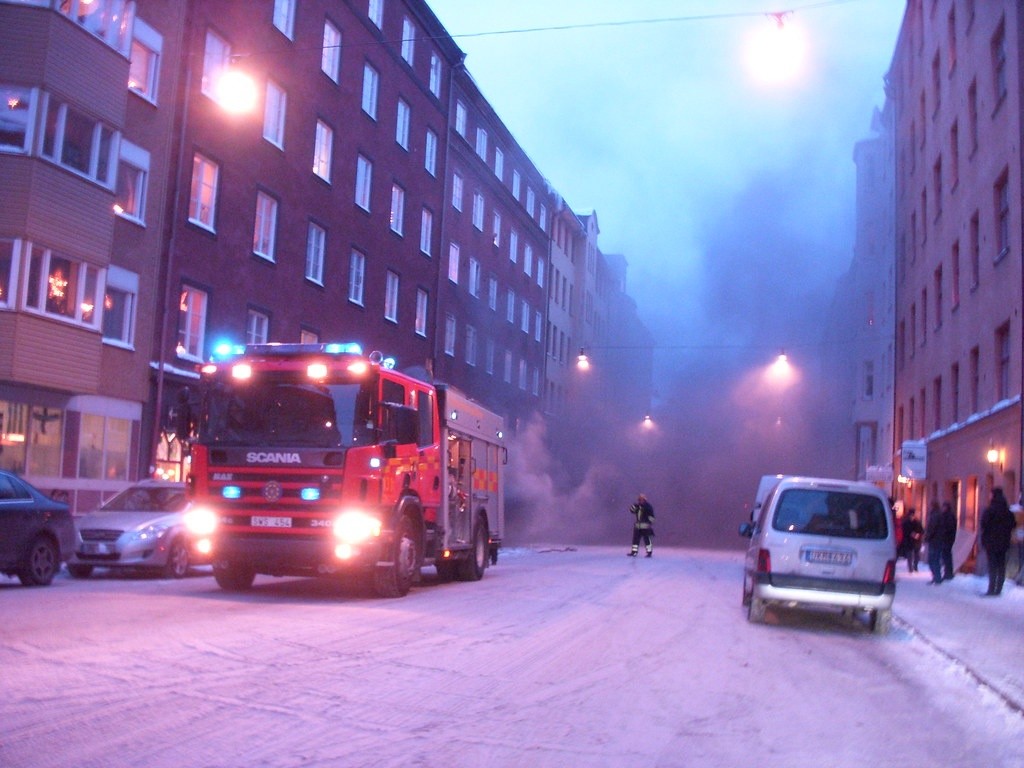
[
  {"left": 979, "top": 486, "right": 1018, "bottom": 596},
  {"left": 627, "top": 493, "right": 656, "bottom": 558},
  {"left": 902, "top": 506, "right": 925, "bottom": 572},
  {"left": 920, "top": 501, "right": 957, "bottom": 584}
]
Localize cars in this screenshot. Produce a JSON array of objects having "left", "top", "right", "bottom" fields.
[
  {"left": 65, "top": 478, "right": 213, "bottom": 578},
  {"left": 0, "top": 469, "right": 74, "bottom": 585}
]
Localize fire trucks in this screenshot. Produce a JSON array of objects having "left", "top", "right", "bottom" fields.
[{"left": 175, "top": 342, "right": 507, "bottom": 598}]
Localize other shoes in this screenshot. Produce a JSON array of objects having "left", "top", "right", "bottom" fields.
[
  {"left": 646, "top": 552, "right": 652, "bottom": 557},
  {"left": 627, "top": 553, "right": 636, "bottom": 556}
]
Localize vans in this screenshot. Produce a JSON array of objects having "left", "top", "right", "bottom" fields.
[{"left": 739, "top": 475, "right": 896, "bottom": 634}]
